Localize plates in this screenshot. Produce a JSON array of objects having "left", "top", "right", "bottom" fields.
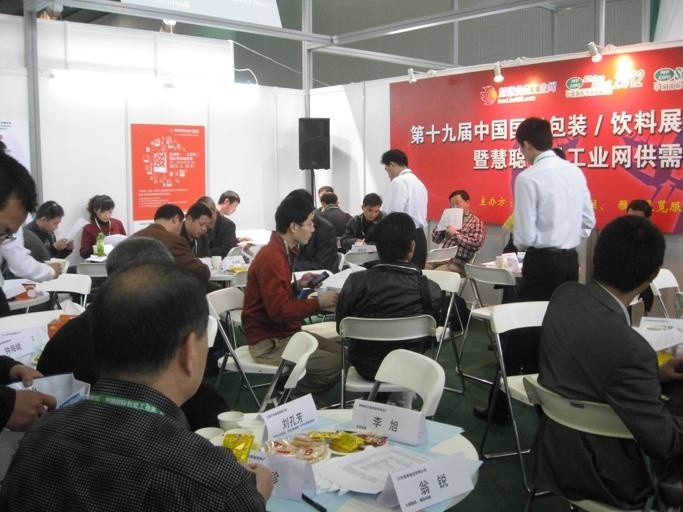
[
  {"left": 312, "top": 452, "right": 332, "bottom": 466},
  {"left": 329, "top": 442, "right": 390, "bottom": 456}
]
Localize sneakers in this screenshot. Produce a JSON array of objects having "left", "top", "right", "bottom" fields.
[{"left": 274, "top": 375, "right": 291, "bottom": 403}]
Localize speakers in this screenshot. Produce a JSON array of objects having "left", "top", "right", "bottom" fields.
[{"left": 299, "top": 119, "right": 329, "bottom": 170}]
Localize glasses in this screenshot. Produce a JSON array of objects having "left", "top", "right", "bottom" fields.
[{"left": 0, "top": 231, "right": 17, "bottom": 244}]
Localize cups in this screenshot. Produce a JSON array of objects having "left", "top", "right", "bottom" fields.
[
  {"left": 193, "top": 410, "right": 267, "bottom": 454},
  {"left": 351, "top": 241, "right": 377, "bottom": 252},
  {"left": 212, "top": 255, "right": 222, "bottom": 270},
  {"left": 495, "top": 256, "right": 504, "bottom": 269}
]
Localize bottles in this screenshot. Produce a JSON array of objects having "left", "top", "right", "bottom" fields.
[{"left": 96, "top": 231, "right": 105, "bottom": 256}]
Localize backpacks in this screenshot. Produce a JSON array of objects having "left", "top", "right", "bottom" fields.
[{"left": 433, "top": 293, "right": 471, "bottom": 332}]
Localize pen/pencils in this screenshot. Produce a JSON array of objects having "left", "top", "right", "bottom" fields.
[{"left": 302, "top": 494, "right": 327, "bottom": 512}]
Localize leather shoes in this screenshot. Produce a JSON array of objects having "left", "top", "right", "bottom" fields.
[{"left": 470, "top": 405, "right": 511, "bottom": 424}]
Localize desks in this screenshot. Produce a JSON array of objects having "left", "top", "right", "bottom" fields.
[{"left": 0, "top": 287, "right": 50, "bottom": 313}]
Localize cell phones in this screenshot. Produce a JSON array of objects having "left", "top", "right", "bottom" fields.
[{"left": 307, "top": 272, "right": 329, "bottom": 289}]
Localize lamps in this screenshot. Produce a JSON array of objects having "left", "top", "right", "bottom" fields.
[
  {"left": 587, "top": 42, "right": 603, "bottom": 62},
  {"left": 408, "top": 68, "right": 424, "bottom": 84},
  {"left": 493, "top": 61, "right": 504, "bottom": 82}
]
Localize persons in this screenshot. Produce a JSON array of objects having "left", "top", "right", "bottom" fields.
[
  {"left": 0, "top": 150, "right": 56, "bottom": 433},
  {"left": 470, "top": 116, "right": 597, "bottom": 426},
  {"left": 488, "top": 148, "right": 567, "bottom": 352},
  {"left": 376, "top": 149, "right": 430, "bottom": 279},
  {"left": 21, "top": 186, "right": 485, "bottom": 432},
  {"left": 626, "top": 199, "right": 654, "bottom": 312},
  {"left": 0, "top": 261, "right": 275, "bottom": 511},
  {"left": 0, "top": 226, "right": 62, "bottom": 284},
  {"left": 528, "top": 214, "right": 681, "bottom": 511}
]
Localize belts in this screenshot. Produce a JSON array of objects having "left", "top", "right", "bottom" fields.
[{"left": 526, "top": 246, "right": 577, "bottom": 256}]
